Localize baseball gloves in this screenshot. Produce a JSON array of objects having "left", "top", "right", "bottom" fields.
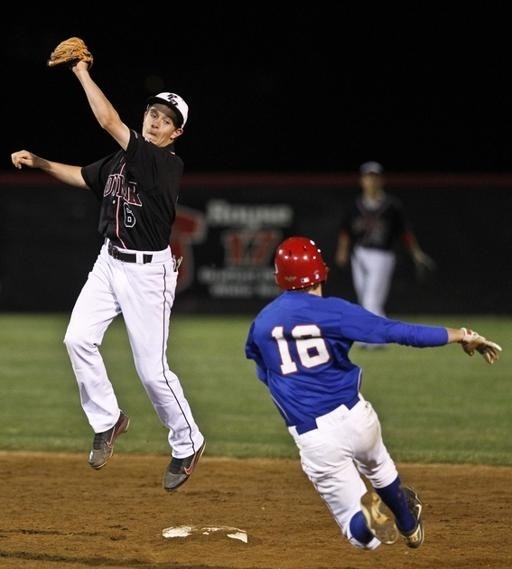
[{"left": 48, "top": 37, "right": 93, "bottom": 76}]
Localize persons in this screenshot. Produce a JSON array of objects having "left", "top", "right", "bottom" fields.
[
  {"left": 11, "top": 37, "right": 206, "bottom": 494},
  {"left": 243, "top": 235, "right": 503, "bottom": 553},
  {"left": 334, "top": 160, "right": 428, "bottom": 352}
]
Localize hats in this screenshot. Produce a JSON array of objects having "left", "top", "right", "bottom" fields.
[
  {"left": 361, "top": 162, "right": 383, "bottom": 179},
  {"left": 147, "top": 91, "right": 188, "bottom": 128}
]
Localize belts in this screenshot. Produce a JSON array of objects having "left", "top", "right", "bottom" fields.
[{"left": 104, "top": 239, "right": 153, "bottom": 265}]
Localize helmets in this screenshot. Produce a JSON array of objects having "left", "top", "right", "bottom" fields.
[{"left": 274, "top": 237, "right": 328, "bottom": 291}]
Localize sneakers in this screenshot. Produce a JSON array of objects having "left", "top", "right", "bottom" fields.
[
  {"left": 164, "top": 439, "right": 206, "bottom": 492},
  {"left": 88, "top": 411, "right": 131, "bottom": 470},
  {"left": 360, "top": 487, "right": 424, "bottom": 549}
]
